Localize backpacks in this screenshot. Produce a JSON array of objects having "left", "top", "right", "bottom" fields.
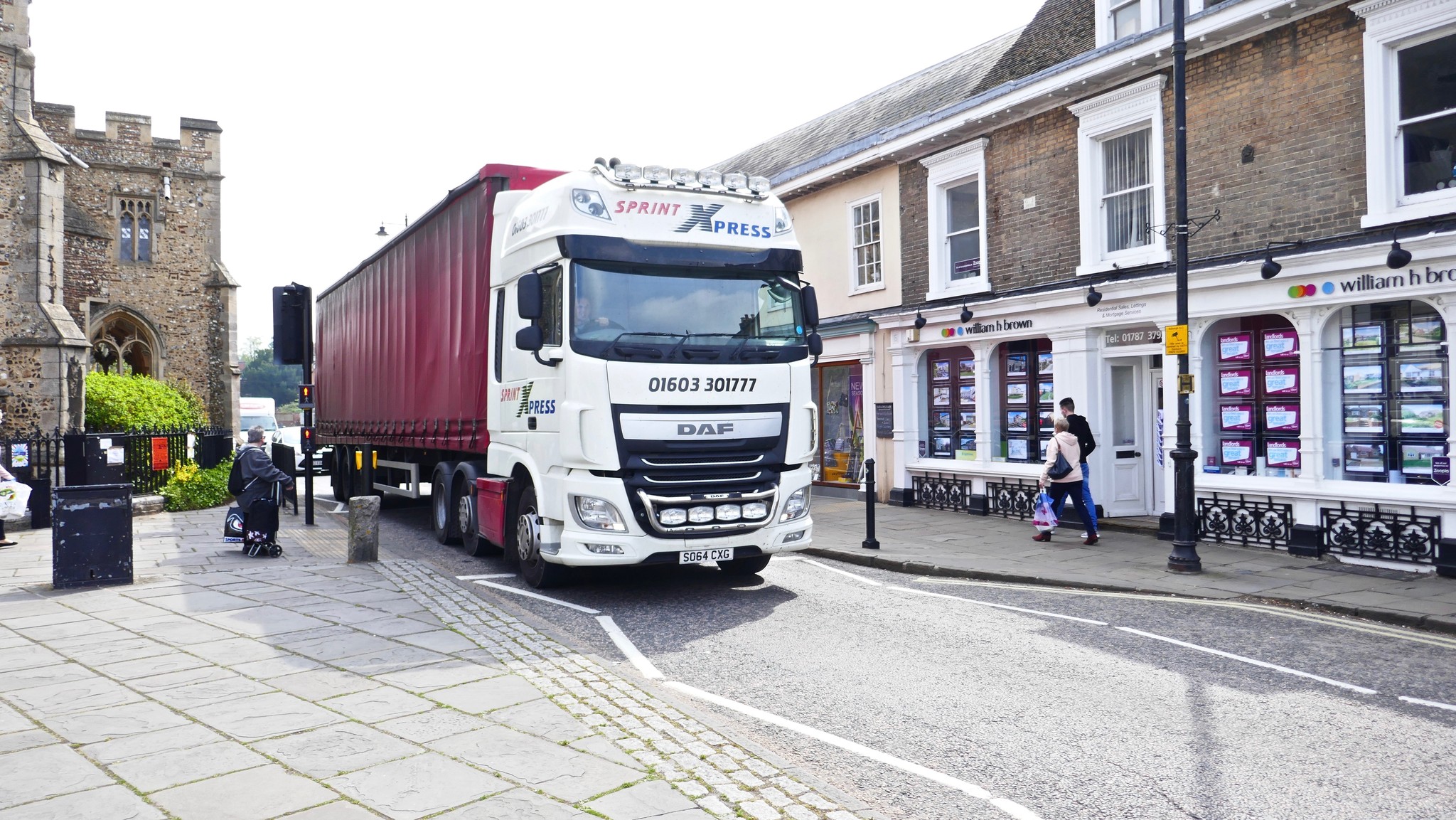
[{"left": 228, "top": 447, "right": 263, "bottom": 494}]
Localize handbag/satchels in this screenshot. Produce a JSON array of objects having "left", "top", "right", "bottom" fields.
[
  {"left": 1033, "top": 487, "right": 1059, "bottom": 532},
  {"left": 223, "top": 507, "right": 245, "bottom": 543},
  {"left": 1047, "top": 437, "right": 1073, "bottom": 480}
]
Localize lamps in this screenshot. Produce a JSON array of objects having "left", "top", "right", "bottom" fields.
[
  {"left": 960, "top": 291, "right": 995, "bottom": 323},
  {"left": 1087, "top": 272, "right": 1119, "bottom": 307},
  {"left": 915, "top": 299, "right": 949, "bottom": 330},
  {"left": 1261, "top": 239, "right": 1303, "bottom": 279},
  {"left": 1386, "top": 219, "right": 1433, "bottom": 268}
]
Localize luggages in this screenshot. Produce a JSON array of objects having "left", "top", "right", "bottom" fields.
[{"left": 245, "top": 481, "right": 282, "bottom": 557}]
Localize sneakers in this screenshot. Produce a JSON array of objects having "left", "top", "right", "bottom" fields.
[
  {"left": 1081, "top": 532, "right": 1100, "bottom": 538},
  {"left": 1051, "top": 531, "right": 1055, "bottom": 534}
]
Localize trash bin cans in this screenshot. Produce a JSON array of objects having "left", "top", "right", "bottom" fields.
[{"left": 29, "top": 478, "right": 52, "bottom": 529}]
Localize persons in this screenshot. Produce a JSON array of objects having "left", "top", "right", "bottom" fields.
[
  {"left": 1050, "top": 397, "right": 1100, "bottom": 538},
  {"left": 233, "top": 424, "right": 294, "bottom": 556},
  {"left": 0, "top": 464, "right": 19, "bottom": 549},
  {"left": 1032, "top": 417, "right": 1098, "bottom": 545}
]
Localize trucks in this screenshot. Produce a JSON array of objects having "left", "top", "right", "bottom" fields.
[{"left": 238, "top": 396, "right": 280, "bottom": 456}]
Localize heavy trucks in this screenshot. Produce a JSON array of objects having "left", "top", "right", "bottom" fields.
[{"left": 316, "top": 159, "right": 824, "bottom": 577}]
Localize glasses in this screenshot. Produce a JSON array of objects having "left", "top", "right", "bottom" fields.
[{"left": 260, "top": 436, "right": 266, "bottom": 439}]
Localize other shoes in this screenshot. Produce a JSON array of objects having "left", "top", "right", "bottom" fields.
[
  {"left": 1082, "top": 535, "right": 1098, "bottom": 545},
  {"left": 1032, "top": 533, "right": 1051, "bottom": 542},
  {"left": 0, "top": 541, "right": 18, "bottom": 547}
]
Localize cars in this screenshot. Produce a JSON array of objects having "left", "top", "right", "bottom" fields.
[{"left": 269, "top": 426, "right": 330, "bottom": 472}]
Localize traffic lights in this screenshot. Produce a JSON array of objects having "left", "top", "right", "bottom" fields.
[
  {"left": 300, "top": 426, "right": 317, "bottom": 452},
  {"left": 299, "top": 384, "right": 316, "bottom": 409}
]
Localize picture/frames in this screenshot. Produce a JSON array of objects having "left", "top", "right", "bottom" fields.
[
  {"left": 1394, "top": 316, "right": 1449, "bottom": 479},
  {"left": 932, "top": 359, "right": 951, "bottom": 383},
  {"left": 932, "top": 435, "right": 952, "bottom": 458},
  {"left": 958, "top": 357, "right": 975, "bottom": 382},
  {"left": 959, "top": 436, "right": 976, "bottom": 450},
  {"left": 1339, "top": 321, "right": 1389, "bottom": 477},
  {"left": 1005, "top": 353, "right": 1029, "bottom": 379},
  {"left": 932, "top": 409, "right": 952, "bottom": 433},
  {"left": 958, "top": 384, "right": 975, "bottom": 408},
  {"left": 1006, "top": 408, "right": 1029, "bottom": 434},
  {"left": 958, "top": 409, "right": 975, "bottom": 434},
  {"left": 1037, "top": 351, "right": 1054, "bottom": 463},
  {"left": 1005, "top": 381, "right": 1029, "bottom": 407},
  {"left": 1007, "top": 436, "right": 1030, "bottom": 462},
  {"left": 932, "top": 384, "right": 952, "bottom": 408}
]
